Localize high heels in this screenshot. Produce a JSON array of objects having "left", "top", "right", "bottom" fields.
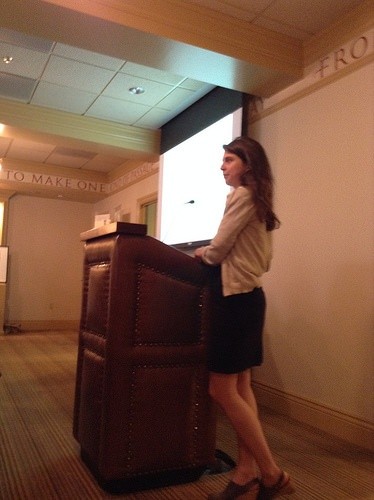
[
  {"left": 206, "top": 475, "right": 261, "bottom": 500},
  {"left": 258, "top": 470, "right": 296, "bottom": 500}
]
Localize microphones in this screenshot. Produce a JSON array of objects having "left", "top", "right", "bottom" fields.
[{"left": 188, "top": 200, "right": 194, "bottom": 204}]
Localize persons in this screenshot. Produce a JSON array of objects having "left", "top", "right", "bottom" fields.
[{"left": 194, "top": 135, "right": 297, "bottom": 500}]
